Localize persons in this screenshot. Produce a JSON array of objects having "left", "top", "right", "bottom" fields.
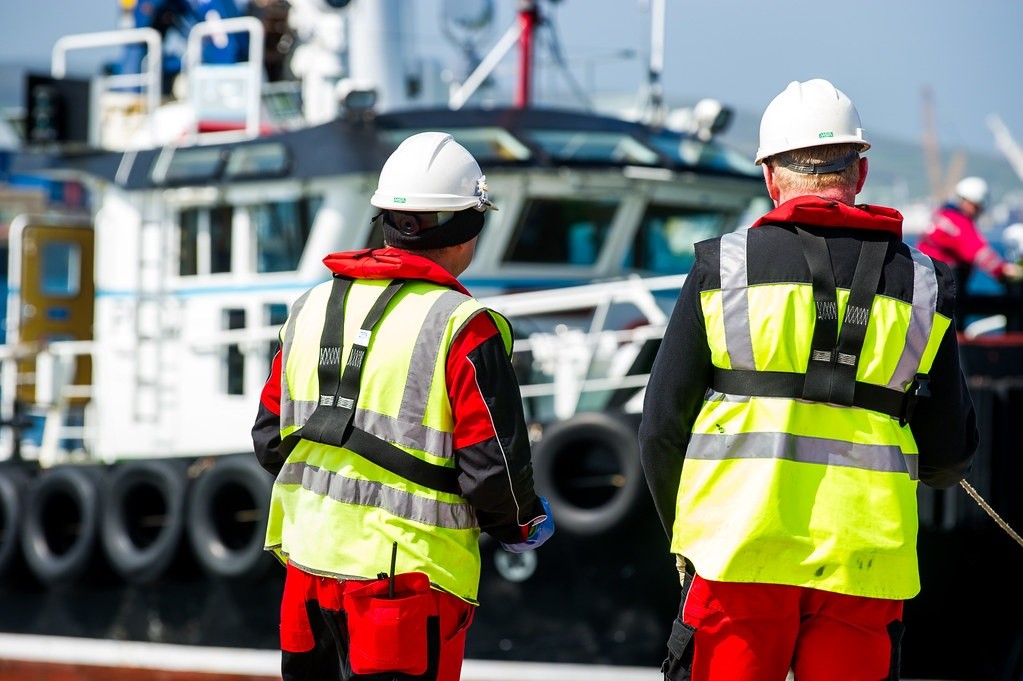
[
  {"left": 637, "top": 78, "right": 979, "bottom": 681},
  {"left": 918, "top": 177, "right": 1023, "bottom": 286},
  {"left": 251, "top": 132, "right": 556, "bottom": 681}
]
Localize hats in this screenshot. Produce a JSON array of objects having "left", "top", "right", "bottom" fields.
[{"left": 382, "top": 206, "right": 484, "bottom": 250}]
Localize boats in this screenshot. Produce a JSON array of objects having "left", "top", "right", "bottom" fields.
[{"left": 0, "top": 1, "right": 1022, "bottom": 662}]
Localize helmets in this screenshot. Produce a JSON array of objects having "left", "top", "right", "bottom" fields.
[
  {"left": 370, "top": 131, "right": 497, "bottom": 212},
  {"left": 756, "top": 78, "right": 872, "bottom": 162},
  {"left": 957, "top": 176, "right": 989, "bottom": 208}
]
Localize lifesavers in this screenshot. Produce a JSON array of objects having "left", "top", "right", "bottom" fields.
[
  {"left": 97, "top": 458, "right": 192, "bottom": 582},
  {"left": 531, "top": 408, "right": 645, "bottom": 542},
  {"left": 21, "top": 462, "right": 111, "bottom": 585},
  {"left": 0, "top": 467, "right": 32, "bottom": 578},
  {"left": 180, "top": 457, "right": 275, "bottom": 588}
]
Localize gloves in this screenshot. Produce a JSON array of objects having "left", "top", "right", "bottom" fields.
[{"left": 502, "top": 498, "right": 555, "bottom": 553}]
